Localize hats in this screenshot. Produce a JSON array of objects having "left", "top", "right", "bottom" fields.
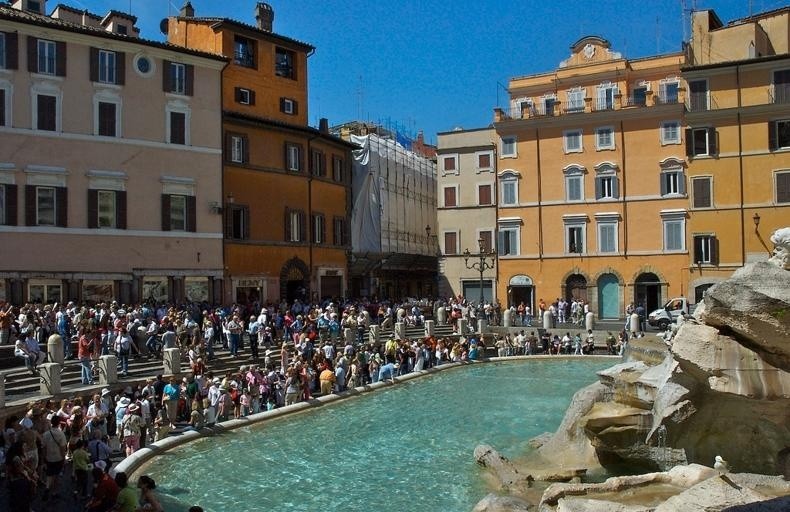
[
  {"left": 265, "top": 326, "right": 271, "bottom": 331},
  {"left": 101, "top": 388, "right": 110, "bottom": 397},
  {"left": 265, "top": 349, "right": 272, "bottom": 355},
  {"left": 95, "top": 460, "right": 107, "bottom": 471},
  {"left": 117, "top": 397, "right": 140, "bottom": 411},
  {"left": 23, "top": 418, "right": 33, "bottom": 429},
  {"left": 213, "top": 377, "right": 227, "bottom": 390},
  {"left": 261, "top": 307, "right": 268, "bottom": 314},
  {"left": 7, "top": 429, "right": 15, "bottom": 434}
]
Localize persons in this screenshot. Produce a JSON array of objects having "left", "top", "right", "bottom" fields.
[{"left": 1, "top": 293, "right": 687, "bottom": 511}]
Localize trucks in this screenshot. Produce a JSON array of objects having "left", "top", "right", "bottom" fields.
[{"left": 648, "top": 297, "right": 698, "bottom": 330}]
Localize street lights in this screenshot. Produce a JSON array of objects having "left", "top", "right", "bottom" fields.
[{"left": 464, "top": 238, "right": 495, "bottom": 320}]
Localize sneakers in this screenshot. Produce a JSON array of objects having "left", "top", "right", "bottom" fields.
[
  {"left": 89, "top": 381, "right": 100, "bottom": 384},
  {"left": 118, "top": 360, "right": 122, "bottom": 365},
  {"left": 122, "top": 370, "right": 126, "bottom": 376},
  {"left": 230, "top": 355, "right": 237, "bottom": 358},
  {"left": 169, "top": 421, "right": 176, "bottom": 429},
  {"left": 27, "top": 369, "right": 39, "bottom": 375},
  {"left": 64, "top": 354, "right": 74, "bottom": 359}
]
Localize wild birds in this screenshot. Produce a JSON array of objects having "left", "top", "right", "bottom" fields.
[{"left": 714, "top": 455, "right": 736, "bottom": 476}]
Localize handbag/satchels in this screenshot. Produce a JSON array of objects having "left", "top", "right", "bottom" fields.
[
  {"left": 120, "top": 348, "right": 130, "bottom": 356},
  {"left": 347, "top": 380, "right": 353, "bottom": 387},
  {"left": 231, "top": 389, "right": 238, "bottom": 401}
]
